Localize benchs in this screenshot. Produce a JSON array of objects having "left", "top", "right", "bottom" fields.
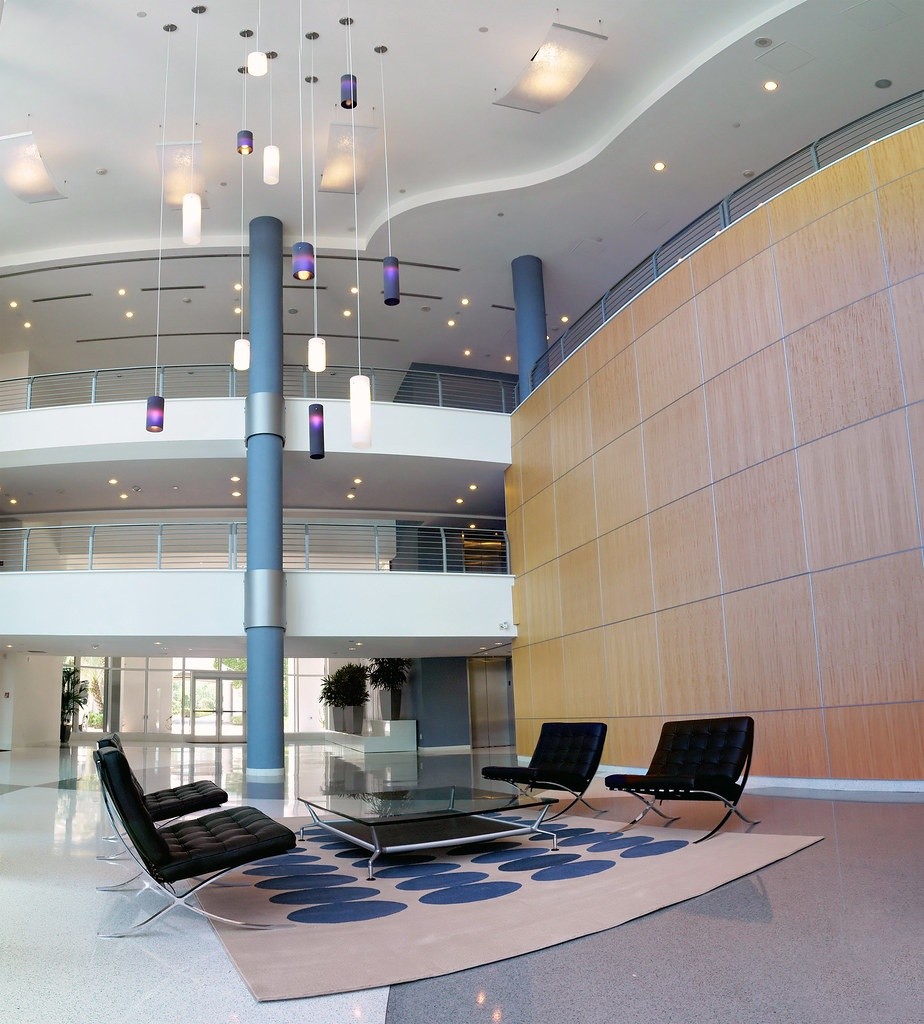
[
  {"left": 605, "top": 716, "right": 762, "bottom": 844},
  {"left": 481, "top": 722, "right": 608, "bottom": 822},
  {"left": 92, "top": 733, "right": 296, "bottom": 937}
]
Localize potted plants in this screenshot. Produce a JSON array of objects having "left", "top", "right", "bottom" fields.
[
  {"left": 60, "top": 667, "right": 89, "bottom": 743},
  {"left": 319, "top": 663, "right": 371, "bottom": 734},
  {"left": 366, "top": 658, "right": 416, "bottom": 720}
]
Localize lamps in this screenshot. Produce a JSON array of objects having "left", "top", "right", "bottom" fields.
[
  {"left": 339, "top": 16, "right": 357, "bottom": 109},
  {"left": 263, "top": 50, "right": 279, "bottom": 185},
  {"left": 350, "top": 0, "right": 371, "bottom": 449},
  {"left": 492, "top": 9, "right": 608, "bottom": 114},
  {"left": 156, "top": 125, "right": 210, "bottom": 209},
  {"left": 234, "top": 65, "right": 250, "bottom": 370},
  {"left": 305, "top": 75, "right": 324, "bottom": 459},
  {"left": 237, "top": 29, "right": 253, "bottom": 155},
  {"left": 305, "top": 31, "right": 326, "bottom": 372},
  {"left": 146, "top": 24, "right": 177, "bottom": 432},
  {"left": 293, "top": 0, "right": 314, "bottom": 280},
  {"left": 318, "top": 105, "right": 380, "bottom": 194},
  {"left": 0, "top": 115, "right": 69, "bottom": 204},
  {"left": 182, "top": 6, "right": 206, "bottom": 246},
  {"left": 248, "top": 0, "right": 267, "bottom": 76},
  {"left": 374, "top": 46, "right": 400, "bottom": 306}
]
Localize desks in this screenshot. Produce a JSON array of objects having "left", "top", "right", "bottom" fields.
[
  {"left": 186, "top": 809, "right": 824, "bottom": 1002},
  {"left": 297, "top": 786, "right": 559, "bottom": 880}
]
